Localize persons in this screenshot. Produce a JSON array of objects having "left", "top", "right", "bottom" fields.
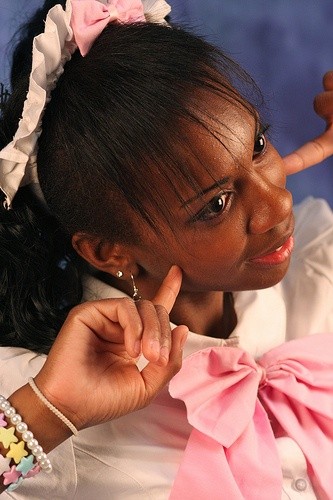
[{"left": 0, "top": 0, "right": 333, "bottom": 500}]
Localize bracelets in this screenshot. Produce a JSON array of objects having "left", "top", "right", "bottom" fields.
[
  {"left": 0, "top": 395, "right": 54, "bottom": 491},
  {"left": 26, "top": 374, "right": 80, "bottom": 437}
]
